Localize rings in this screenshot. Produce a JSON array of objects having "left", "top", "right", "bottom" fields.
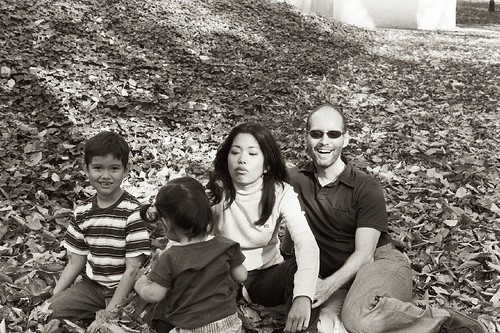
[{"left": 297, "top": 324, "right": 302, "bottom": 327}]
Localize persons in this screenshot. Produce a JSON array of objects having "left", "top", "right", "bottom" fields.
[
  {"left": 37, "top": 130, "right": 152, "bottom": 332},
  {"left": 280, "top": 105, "right": 490, "bottom": 333},
  {"left": 135, "top": 178, "right": 247, "bottom": 333},
  {"left": 210, "top": 121, "right": 321, "bottom": 333}
]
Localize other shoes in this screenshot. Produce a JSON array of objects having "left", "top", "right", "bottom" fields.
[{"left": 438, "top": 306, "right": 487, "bottom": 333}]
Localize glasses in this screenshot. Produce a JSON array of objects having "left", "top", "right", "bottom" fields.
[{"left": 306, "top": 129, "right": 344, "bottom": 139}]
